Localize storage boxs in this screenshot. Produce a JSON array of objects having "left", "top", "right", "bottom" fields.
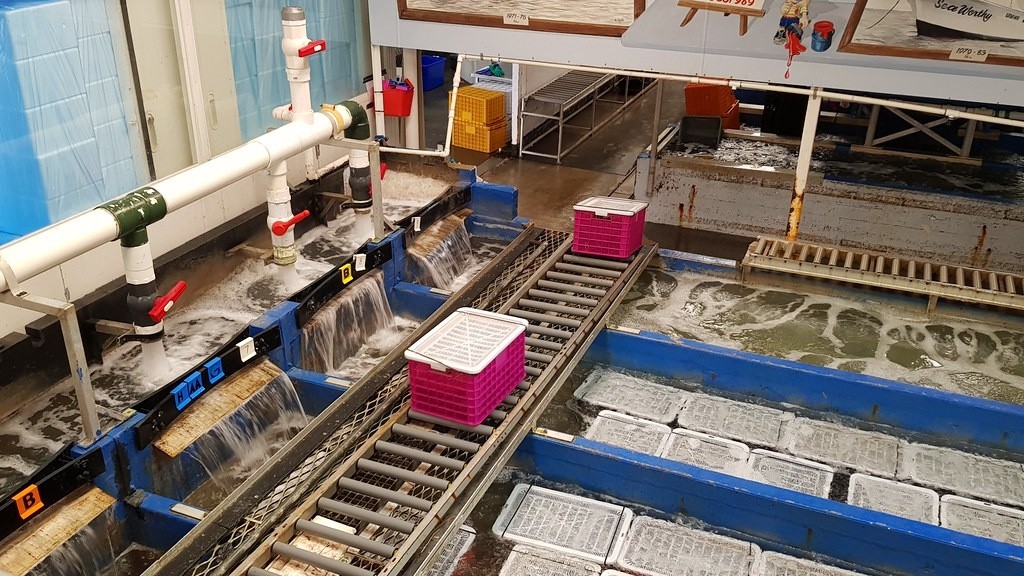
[
  {"left": 421, "top": 55, "right": 447, "bottom": 91},
  {"left": 571, "top": 196, "right": 649, "bottom": 259},
  {"left": 404, "top": 306, "right": 527, "bottom": 427},
  {"left": 447, "top": 81, "right": 512, "bottom": 153},
  {"left": 761, "top": 88, "right": 809, "bottom": 137},
  {"left": 373, "top": 79, "right": 415, "bottom": 117},
  {"left": 677, "top": 83, "right": 741, "bottom": 151},
  {"left": 363, "top": 369, "right": 1024, "bottom": 576}
]
